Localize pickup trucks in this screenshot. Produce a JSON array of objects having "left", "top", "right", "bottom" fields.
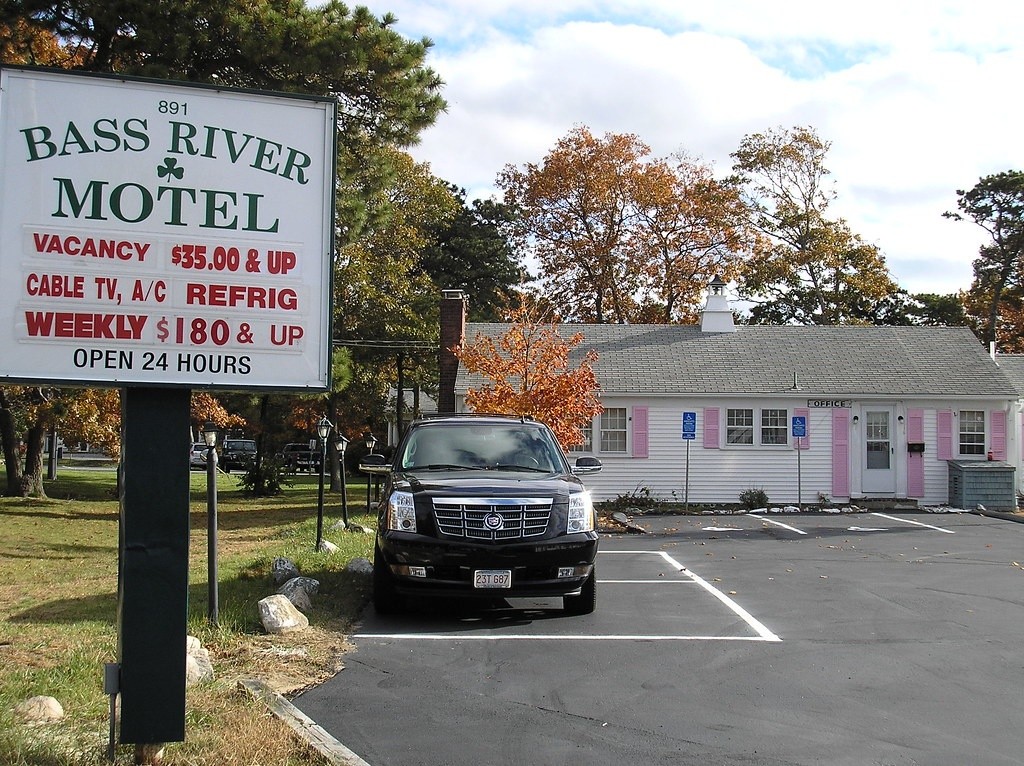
[{"left": 272, "top": 442, "right": 322, "bottom": 474}]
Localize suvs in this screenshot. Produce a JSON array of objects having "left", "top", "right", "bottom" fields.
[
  {"left": 356, "top": 410, "right": 605, "bottom": 618},
  {"left": 190, "top": 442, "right": 209, "bottom": 470},
  {"left": 216, "top": 440, "right": 259, "bottom": 474}
]
